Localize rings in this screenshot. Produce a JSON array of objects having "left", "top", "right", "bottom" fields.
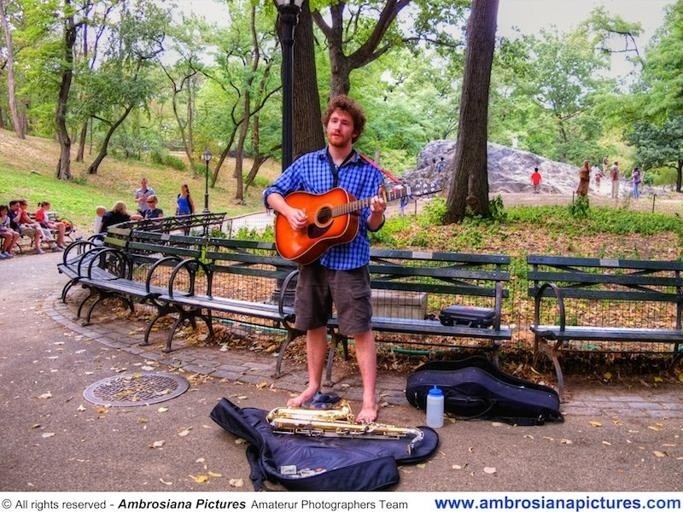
[{"left": 378, "top": 206, "right": 382, "bottom": 210}]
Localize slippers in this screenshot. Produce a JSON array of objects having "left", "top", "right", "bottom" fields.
[{"left": 302, "top": 390, "right": 340, "bottom": 410}]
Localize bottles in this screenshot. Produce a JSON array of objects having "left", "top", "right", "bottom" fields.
[{"left": 426, "top": 383, "right": 445, "bottom": 428}]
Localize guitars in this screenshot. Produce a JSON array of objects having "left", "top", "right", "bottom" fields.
[{"left": 275, "top": 179, "right": 441, "bottom": 267}]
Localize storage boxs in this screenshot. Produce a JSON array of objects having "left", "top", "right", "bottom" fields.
[{"left": 369, "top": 289, "right": 427, "bottom": 320}]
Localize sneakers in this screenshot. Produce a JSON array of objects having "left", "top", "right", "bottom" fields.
[{"left": 0, "top": 243, "right": 69, "bottom": 259}]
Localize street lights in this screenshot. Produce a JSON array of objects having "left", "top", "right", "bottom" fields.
[{"left": 201, "top": 149, "right": 211, "bottom": 227}]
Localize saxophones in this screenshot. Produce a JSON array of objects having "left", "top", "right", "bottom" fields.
[{"left": 266, "top": 400, "right": 424, "bottom": 455}]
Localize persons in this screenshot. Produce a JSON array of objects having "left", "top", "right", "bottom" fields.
[
  {"left": 0, "top": 177, "right": 194, "bottom": 258},
  {"left": 530, "top": 168, "right": 541, "bottom": 192},
  {"left": 574, "top": 155, "right": 640, "bottom": 199},
  {"left": 263, "top": 95, "right": 388, "bottom": 424}
]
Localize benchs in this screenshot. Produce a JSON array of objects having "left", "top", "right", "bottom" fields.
[
  {"left": 0, "top": 212, "right": 75, "bottom": 254},
  {"left": 60, "top": 215, "right": 305, "bottom": 379},
  {"left": 526, "top": 250, "right": 683, "bottom": 403},
  {"left": 326, "top": 247, "right": 513, "bottom": 386}
]
